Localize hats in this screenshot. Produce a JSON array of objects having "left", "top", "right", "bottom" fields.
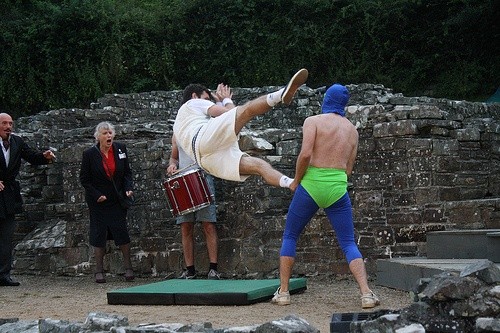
[{"left": 322, "top": 85, "right": 349, "bottom": 117}]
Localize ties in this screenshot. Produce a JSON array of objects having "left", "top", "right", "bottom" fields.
[{"left": 3, "top": 140, "right": 10, "bottom": 152}]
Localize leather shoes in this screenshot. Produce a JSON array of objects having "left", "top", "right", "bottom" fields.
[{"left": 0, "top": 278, "right": 20, "bottom": 286}]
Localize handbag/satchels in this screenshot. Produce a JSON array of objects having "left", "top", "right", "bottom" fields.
[{"left": 118, "top": 191, "right": 134, "bottom": 209}]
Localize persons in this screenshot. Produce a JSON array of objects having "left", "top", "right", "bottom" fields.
[
  {"left": 270, "top": 84, "right": 382, "bottom": 308},
  {"left": 79, "top": 122, "right": 135, "bottom": 284},
  {"left": 166, "top": 131, "right": 222, "bottom": 279},
  {"left": 0, "top": 113, "right": 55, "bottom": 286},
  {"left": 172, "top": 67, "right": 309, "bottom": 188}
]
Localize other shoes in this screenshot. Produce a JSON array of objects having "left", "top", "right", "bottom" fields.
[
  {"left": 127, "top": 275, "right": 134, "bottom": 280},
  {"left": 96, "top": 272, "right": 106, "bottom": 283},
  {"left": 181, "top": 272, "right": 196, "bottom": 278},
  {"left": 208, "top": 274, "right": 221, "bottom": 279},
  {"left": 281, "top": 69, "right": 309, "bottom": 105}
]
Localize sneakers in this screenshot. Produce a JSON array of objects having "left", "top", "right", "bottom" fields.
[
  {"left": 362, "top": 290, "right": 380, "bottom": 307},
  {"left": 272, "top": 287, "right": 290, "bottom": 305}
]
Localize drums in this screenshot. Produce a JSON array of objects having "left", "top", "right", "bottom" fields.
[{"left": 164, "top": 168, "right": 214, "bottom": 217}]
